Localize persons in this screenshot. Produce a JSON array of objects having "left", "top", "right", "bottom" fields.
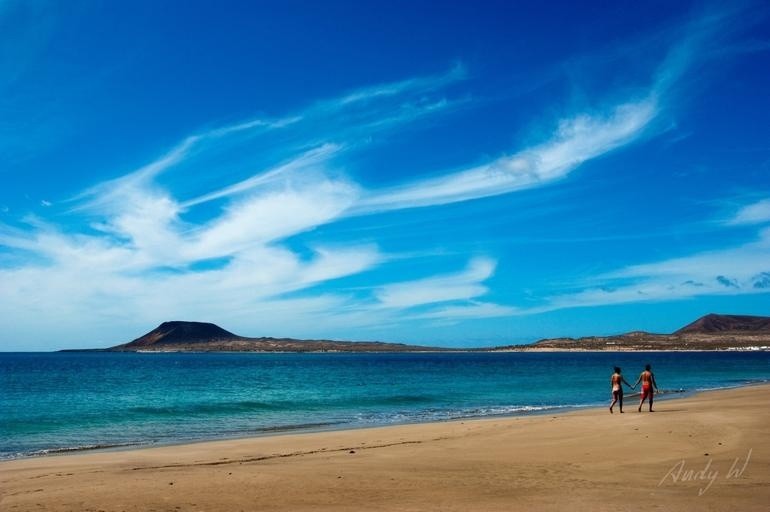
[
  {"left": 634, "top": 365, "right": 658, "bottom": 412},
  {"left": 609, "top": 367, "right": 634, "bottom": 413}
]
